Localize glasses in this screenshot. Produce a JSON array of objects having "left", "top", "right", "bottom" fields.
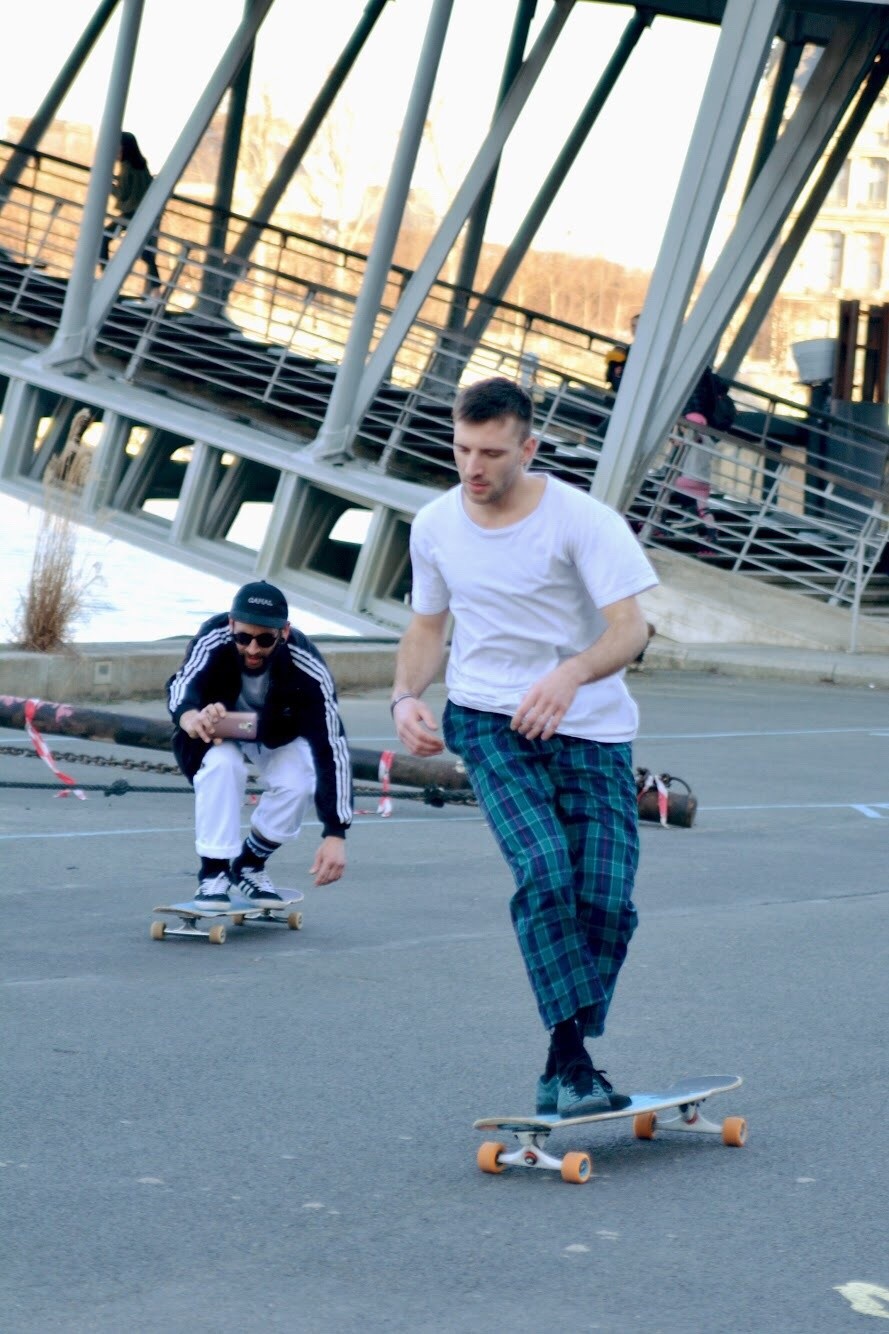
[{"left": 232, "top": 632, "right": 276, "bottom": 647}]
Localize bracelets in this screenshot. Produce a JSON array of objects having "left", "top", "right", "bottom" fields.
[{"left": 390, "top": 695, "right": 413, "bottom": 720}]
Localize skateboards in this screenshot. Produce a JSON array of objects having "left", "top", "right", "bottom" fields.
[
  {"left": 150, "top": 888, "right": 304, "bottom": 945},
  {"left": 472, "top": 1074, "right": 747, "bottom": 1184}
]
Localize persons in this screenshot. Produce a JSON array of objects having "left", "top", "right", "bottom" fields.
[
  {"left": 389, "top": 376, "right": 662, "bottom": 1121},
  {"left": 99, "top": 132, "right": 163, "bottom": 307},
  {"left": 669, "top": 366, "right": 736, "bottom": 556},
  {"left": 164, "top": 580, "right": 354, "bottom": 911},
  {"left": 590, "top": 315, "right": 641, "bottom": 449}
]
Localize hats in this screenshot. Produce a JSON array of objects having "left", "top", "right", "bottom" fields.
[{"left": 231, "top": 580, "right": 288, "bottom": 628}]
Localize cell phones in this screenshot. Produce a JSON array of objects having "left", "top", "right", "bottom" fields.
[{"left": 205, "top": 711, "right": 257, "bottom": 739}]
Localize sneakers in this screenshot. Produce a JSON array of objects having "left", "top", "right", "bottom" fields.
[
  {"left": 535, "top": 1068, "right": 632, "bottom": 1113},
  {"left": 230, "top": 856, "right": 282, "bottom": 906},
  {"left": 556, "top": 1060, "right": 610, "bottom": 1119},
  {"left": 192, "top": 871, "right": 233, "bottom": 911}
]
